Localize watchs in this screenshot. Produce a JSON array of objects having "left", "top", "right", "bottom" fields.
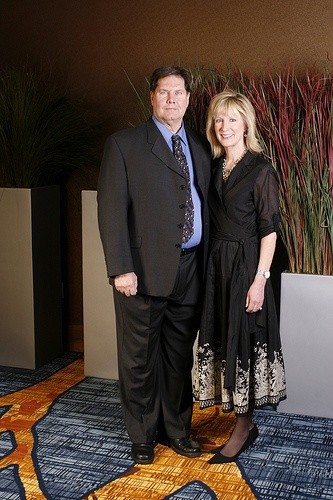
[{"left": 255, "top": 270, "right": 272, "bottom": 279}]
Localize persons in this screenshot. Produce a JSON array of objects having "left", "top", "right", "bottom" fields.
[
  {"left": 96, "top": 66, "right": 211, "bottom": 465},
  {"left": 189, "top": 91, "right": 289, "bottom": 465}
]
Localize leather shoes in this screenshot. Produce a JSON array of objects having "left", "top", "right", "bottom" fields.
[
  {"left": 169, "top": 437, "right": 202, "bottom": 457},
  {"left": 131, "top": 443, "right": 154, "bottom": 464}
]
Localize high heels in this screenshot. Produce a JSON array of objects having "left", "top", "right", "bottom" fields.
[
  {"left": 210, "top": 431, "right": 233, "bottom": 454},
  {"left": 208, "top": 423, "right": 258, "bottom": 464}
]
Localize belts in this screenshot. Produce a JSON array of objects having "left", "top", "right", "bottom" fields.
[{"left": 181, "top": 246, "right": 199, "bottom": 254}]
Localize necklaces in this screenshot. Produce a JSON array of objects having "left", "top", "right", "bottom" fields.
[{"left": 221, "top": 148, "right": 248, "bottom": 178}]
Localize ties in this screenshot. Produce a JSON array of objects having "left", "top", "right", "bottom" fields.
[{"left": 171, "top": 135, "right": 194, "bottom": 244}]
